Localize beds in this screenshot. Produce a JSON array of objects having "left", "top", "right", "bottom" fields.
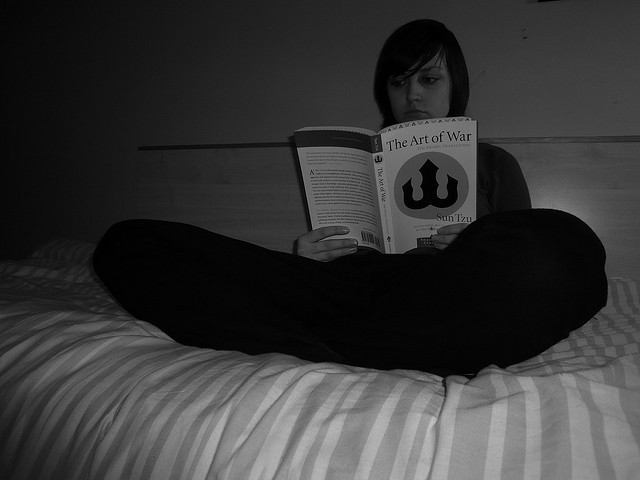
[{"left": 0, "top": 134, "right": 639, "bottom": 480}]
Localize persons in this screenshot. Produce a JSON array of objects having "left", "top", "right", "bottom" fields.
[{"left": 92, "top": 20, "right": 607, "bottom": 377}]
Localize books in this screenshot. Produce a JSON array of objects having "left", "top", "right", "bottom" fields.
[{"left": 287, "top": 116, "right": 479, "bottom": 255}]
